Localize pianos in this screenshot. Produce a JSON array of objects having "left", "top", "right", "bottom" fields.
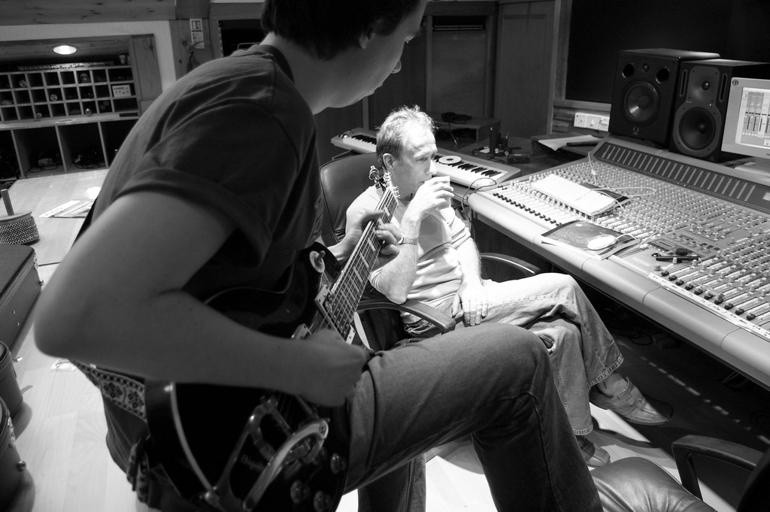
[{"left": 331, "top": 128, "right": 522, "bottom": 190}]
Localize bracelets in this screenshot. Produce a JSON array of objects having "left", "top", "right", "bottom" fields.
[{"left": 396, "top": 236, "right": 421, "bottom": 246}]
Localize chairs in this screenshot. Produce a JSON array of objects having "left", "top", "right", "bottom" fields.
[
  {"left": 588, "top": 433, "right": 770, "bottom": 512},
  {"left": 317, "top": 154, "right": 540, "bottom": 352}
]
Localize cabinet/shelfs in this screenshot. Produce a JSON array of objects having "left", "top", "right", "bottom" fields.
[{"left": 0, "top": 65, "right": 141, "bottom": 179}]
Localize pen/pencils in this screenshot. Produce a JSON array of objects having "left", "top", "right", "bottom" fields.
[{"left": 656, "top": 256, "right": 699, "bottom": 261}]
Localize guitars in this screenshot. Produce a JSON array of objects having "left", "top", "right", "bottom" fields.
[{"left": 144, "top": 167, "right": 401, "bottom": 511}]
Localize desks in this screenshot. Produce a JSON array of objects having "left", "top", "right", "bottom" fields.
[{"left": 430, "top": 112, "right": 501, "bottom": 146}]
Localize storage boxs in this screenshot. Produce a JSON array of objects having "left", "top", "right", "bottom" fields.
[{"left": 0, "top": 241, "right": 44, "bottom": 353}]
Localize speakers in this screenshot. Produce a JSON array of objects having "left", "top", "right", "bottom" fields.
[
  {"left": 667, "top": 58, "right": 770, "bottom": 165},
  {"left": 609, "top": 48, "right": 720, "bottom": 150}
]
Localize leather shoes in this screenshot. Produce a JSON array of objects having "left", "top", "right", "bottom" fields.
[
  {"left": 575, "top": 434, "right": 609, "bottom": 467},
  {"left": 590, "top": 376, "right": 673, "bottom": 426}
]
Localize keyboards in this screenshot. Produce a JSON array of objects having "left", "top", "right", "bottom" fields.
[{"left": 533, "top": 174, "right": 615, "bottom": 217}]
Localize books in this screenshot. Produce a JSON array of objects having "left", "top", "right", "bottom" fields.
[
  {"left": 531, "top": 172, "right": 632, "bottom": 215},
  {"left": 540, "top": 218, "right": 642, "bottom": 260}
]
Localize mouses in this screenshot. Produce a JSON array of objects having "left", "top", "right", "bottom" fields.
[{"left": 588, "top": 233, "right": 618, "bottom": 250}]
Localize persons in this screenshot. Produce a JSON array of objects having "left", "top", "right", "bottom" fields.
[
  {"left": 342, "top": 105, "right": 674, "bottom": 466},
  {"left": 33, "top": 0, "right": 602, "bottom": 511}
]
